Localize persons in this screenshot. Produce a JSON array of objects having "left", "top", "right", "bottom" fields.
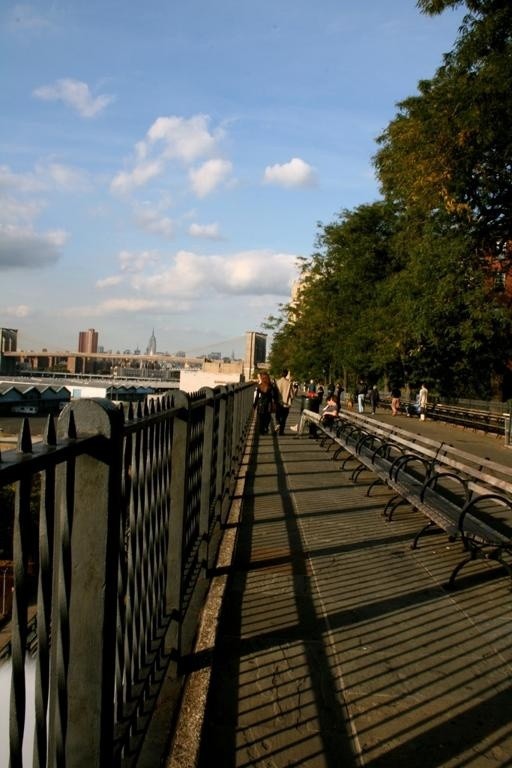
[{"left": 253, "top": 367, "right": 428, "bottom": 434}]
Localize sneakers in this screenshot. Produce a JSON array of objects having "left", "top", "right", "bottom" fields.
[{"left": 290, "top": 424, "right": 298, "bottom": 431}]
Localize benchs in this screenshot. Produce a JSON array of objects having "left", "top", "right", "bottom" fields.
[
  {"left": 355, "top": 389, "right": 511, "bottom": 448},
  {"left": 305, "top": 401, "right": 512, "bottom": 591}
]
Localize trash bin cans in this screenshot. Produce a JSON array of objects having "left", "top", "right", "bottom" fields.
[{"left": 301, "top": 396, "right": 319, "bottom": 414}]
